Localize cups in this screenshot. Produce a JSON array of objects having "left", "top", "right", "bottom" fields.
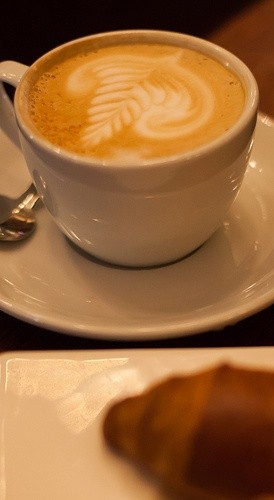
[{"left": 0, "top": 29, "right": 259, "bottom": 268}]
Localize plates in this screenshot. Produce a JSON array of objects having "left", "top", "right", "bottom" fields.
[
  {"left": 0, "top": 110, "right": 274, "bottom": 341},
  {"left": 1, "top": 349, "right": 274, "bottom": 499}
]
